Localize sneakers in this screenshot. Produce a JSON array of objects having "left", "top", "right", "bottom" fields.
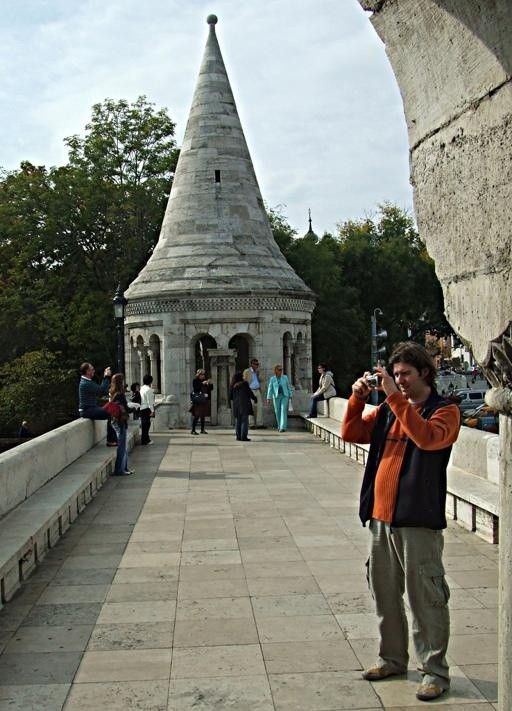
[
  {"left": 191, "top": 430, "right": 207, "bottom": 435},
  {"left": 124, "top": 468, "right": 135, "bottom": 474},
  {"left": 416, "top": 674, "right": 447, "bottom": 700},
  {"left": 107, "top": 442, "right": 118, "bottom": 447},
  {"left": 360, "top": 664, "right": 400, "bottom": 680},
  {"left": 149, "top": 440, "right": 154, "bottom": 445},
  {"left": 237, "top": 438, "right": 251, "bottom": 441},
  {"left": 306, "top": 415, "right": 310, "bottom": 417},
  {"left": 279, "top": 429, "right": 285, "bottom": 432}
]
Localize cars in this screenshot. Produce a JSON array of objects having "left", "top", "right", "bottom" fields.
[{"left": 462, "top": 404, "right": 488, "bottom": 419}]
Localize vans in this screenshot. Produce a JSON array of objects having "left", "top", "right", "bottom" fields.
[{"left": 450, "top": 389, "right": 488, "bottom": 411}]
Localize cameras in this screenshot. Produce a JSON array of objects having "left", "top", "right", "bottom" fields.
[{"left": 366, "top": 374, "right": 381, "bottom": 387}]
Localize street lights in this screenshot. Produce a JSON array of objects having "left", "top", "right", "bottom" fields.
[
  {"left": 112, "top": 283, "right": 127, "bottom": 376},
  {"left": 371, "top": 308, "right": 388, "bottom": 405}
]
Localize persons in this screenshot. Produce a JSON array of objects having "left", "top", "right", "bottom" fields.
[
  {"left": 243, "top": 359, "right": 267, "bottom": 429},
  {"left": 107, "top": 374, "right": 134, "bottom": 475},
  {"left": 340, "top": 339, "right": 462, "bottom": 700},
  {"left": 131, "top": 382, "right": 141, "bottom": 414},
  {"left": 188, "top": 369, "right": 213, "bottom": 434},
  {"left": 229, "top": 373, "right": 257, "bottom": 440},
  {"left": 266, "top": 364, "right": 294, "bottom": 432},
  {"left": 139, "top": 374, "right": 156, "bottom": 445},
  {"left": 307, "top": 363, "right": 337, "bottom": 418},
  {"left": 78, "top": 363, "right": 117, "bottom": 446}
]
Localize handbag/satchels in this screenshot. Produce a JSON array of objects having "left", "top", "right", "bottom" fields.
[
  {"left": 190, "top": 391, "right": 205, "bottom": 404},
  {"left": 288, "top": 398, "right": 294, "bottom": 412},
  {"left": 102, "top": 401, "right": 120, "bottom": 417}
]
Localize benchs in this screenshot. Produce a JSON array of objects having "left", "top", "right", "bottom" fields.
[
  {"left": 288, "top": 391, "right": 500, "bottom": 546},
  {"left": 1, "top": 398, "right": 142, "bottom": 612}
]
